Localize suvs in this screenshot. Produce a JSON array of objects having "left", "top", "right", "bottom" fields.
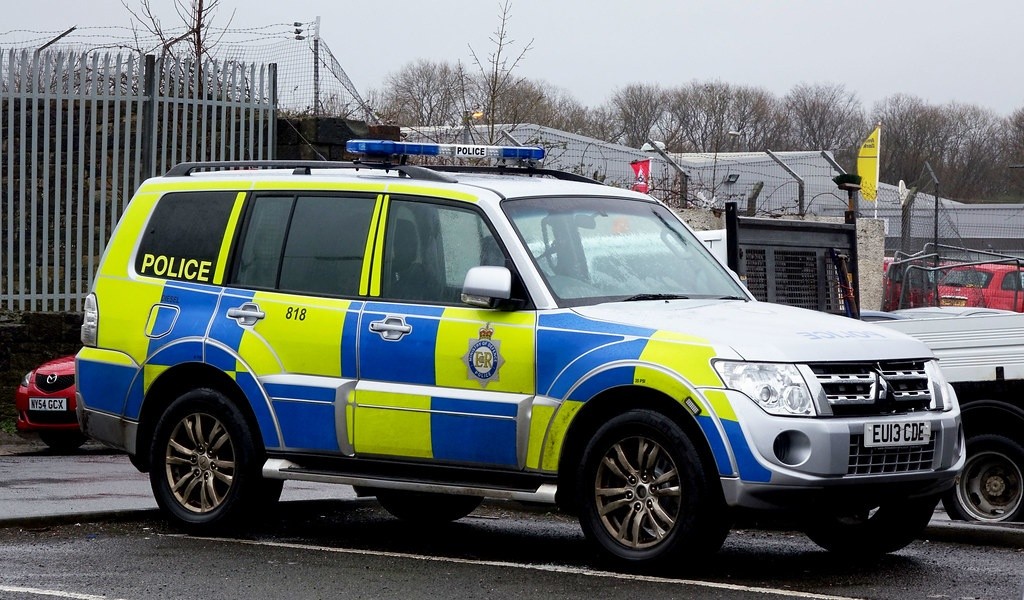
[{"left": 76, "top": 141, "right": 966, "bottom": 576}]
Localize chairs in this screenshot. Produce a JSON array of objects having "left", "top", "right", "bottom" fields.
[
  {"left": 480, "top": 236, "right": 506, "bottom": 268},
  {"left": 392, "top": 218, "right": 428, "bottom": 300}
]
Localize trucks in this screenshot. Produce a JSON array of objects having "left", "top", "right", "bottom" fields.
[{"left": 523, "top": 203, "right": 1024, "bottom": 521}]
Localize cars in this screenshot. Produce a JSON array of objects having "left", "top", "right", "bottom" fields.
[
  {"left": 16, "top": 354, "right": 89, "bottom": 452},
  {"left": 854, "top": 256, "right": 1024, "bottom": 322}
]
